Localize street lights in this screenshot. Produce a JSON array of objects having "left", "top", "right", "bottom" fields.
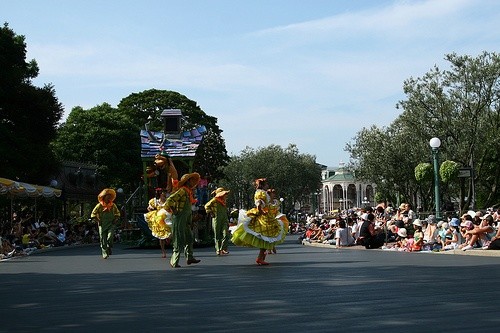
[
  {"left": 338, "top": 199, "right": 342, "bottom": 212},
  {"left": 50, "top": 180, "right": 57, "bottom": 217},
  {"left": 430, "top": 136, "right": 441, "bottom": 221},
  {"left": 280, "top": 197, "right": 284, "bottom": 214},
  {"left": 364, "top": 197, "right": 368, "bottom": 210},
  {"left": 117, "top": 188, "right": 123, "bottom": 206}
]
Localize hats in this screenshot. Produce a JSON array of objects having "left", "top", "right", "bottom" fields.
[
  {"left": 461, "top": 220, "right": 473, "bottom": 228},
  {"left": 448, "top": 218, "right": 459, "bottom": 226},
  {"left": 255, "top": 178, "right": 267, "bottom": 188},
  {"left": 98, "top": 188, "right": 117, "bottom": 202},
  {"left": 177, "top": 172, "right": 200, "bottom": 189},
  {"left": 215, "top": 188, "right": 230, "bottom": 197},
  {"left": 438, "top": 221, "right": 444, "bottom": 226},
  {"left": 412, "top": 219, "right": 423, "bottom": 226},
  {"left": 422, "top": 221, "right": 427, "bottom": 224},
  {"left": 475, "top": 212, "right": 482, "bottom": 217},
  {"left": 399, "top": 203, "right": 405, "bottom": 209},
  {"left": 396, "top": 220, "right": 404, "bottom": 227},
  {"left": 397, "top": 228, "right": 407, "bottom": 237},
  {"left": 479, "top": 214, "right": 493, "bottom": 221},
  {"left": 426, "top": 215, "right": 434, "bottom": 224},
  {"left": 460, "top": 214, "right": 467, "bottom": 218},
  {"left": 360, "top": 213, "right": 368, "bottom": 220}
]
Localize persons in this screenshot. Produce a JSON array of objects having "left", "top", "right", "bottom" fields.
[
  {"left": 143, "top": 172, "right": 230, "bottom": 268},
  {"left": 230, "top": 178, "right": 289, "bottom": 265},
  {"left": 287, "top": 202, "right": 500, "bottom": 253},
  {"left": 0, "top": 209, "right": 120, "bottom": 245},
  {"left": 90, "top": 189, "right": 120, "bottom": 259}
]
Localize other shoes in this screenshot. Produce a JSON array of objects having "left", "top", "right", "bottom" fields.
[
  {"left": 216, "top": 251, "right": 220, "bottom": 255},
  {"left": 103, "top": 253, "right": 109, "bottom": 258},
  {"left": 221, "top": 250, "right": 229, "bottom": 254},
  {"left": 172, "top": 265, "right": 181, "bottom": 268},
  {"left": 162, "top": 256, "right": 166, "bottom": 258},
  {"left": 462, "top": 245, "right": 473, "bottom": 250},
  {"left": 187, "top": 259, "right": 200, "bottom": 264},
  {"left": 109, "top": 250, "right": 112, "bottom": 255}
]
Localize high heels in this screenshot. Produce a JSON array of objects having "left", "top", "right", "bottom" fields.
[{"left": 256, "top": 257, "right": 268, "bottom": 265}]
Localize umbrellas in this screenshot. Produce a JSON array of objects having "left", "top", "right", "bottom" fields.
[{"left": 0, "top": 178, "right": 62, "bottom": 223}]
